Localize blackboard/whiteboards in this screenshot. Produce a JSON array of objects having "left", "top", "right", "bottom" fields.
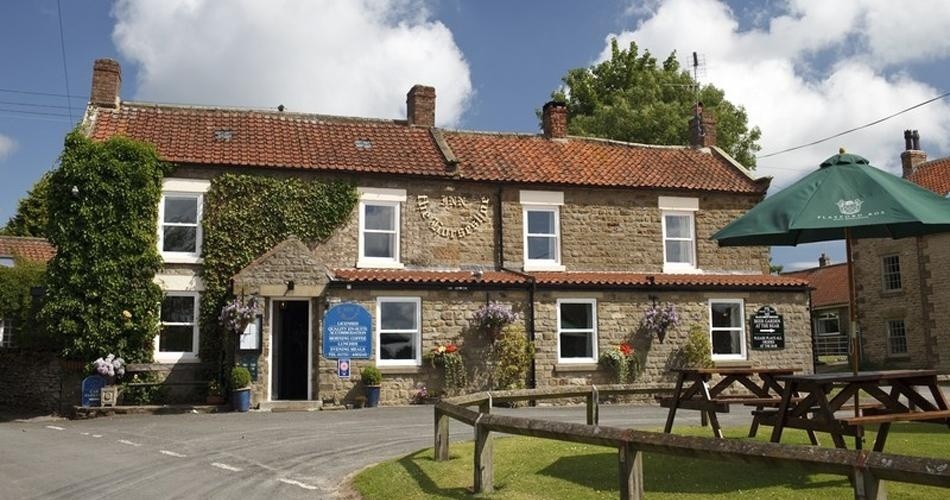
[
  {"left": 322, "top": 303, "right": 371, "bottom": 358},
  {"left": 750, "top": 305, "right": 784, "bottom": 350}
]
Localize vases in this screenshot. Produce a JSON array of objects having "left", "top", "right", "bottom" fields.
[{"left": 206, "top": 395, "right": 225, "bottom": 404}]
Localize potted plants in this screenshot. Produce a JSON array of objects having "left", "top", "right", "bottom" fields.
[
  {"left": 229, "top": 365, "right": 252, "bottom": 411},
  {"left": 355, "top": 364, "right": 382, "bottom": 408}
]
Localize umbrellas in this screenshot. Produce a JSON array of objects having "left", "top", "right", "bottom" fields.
[{"left": 708, "top": 148, "right": 950, "bottom": 417}]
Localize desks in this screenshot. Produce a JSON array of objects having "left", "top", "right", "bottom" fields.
[
  {"left": 663, "top": 366, "right": 821, "bottom": 446},
  {"left": 770, "top": 368, "right": 949, "bottom": 453}
]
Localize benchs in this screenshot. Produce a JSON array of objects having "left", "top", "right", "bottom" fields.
[
  {"left": 654, "top": 392, "right": 820, "bottom": 447},
  {"left": 747, "top": 401, "right": 950, "bottom": 454}
]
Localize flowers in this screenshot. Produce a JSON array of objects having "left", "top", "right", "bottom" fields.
[
  {"left": 217, "top": 299, "right": 259, "bottom": 335},
  {"left": 471, "top": 300, "right": 519, "bottom": 328},
  {"left": 207, "top": 379, "right": 225, "bottom": 397},
  {"left": 641, "top": 301, "right": 678, "bottom": 334},
  {"left": 94, "top": 353, "right": 126, "bottom": 383}
]
